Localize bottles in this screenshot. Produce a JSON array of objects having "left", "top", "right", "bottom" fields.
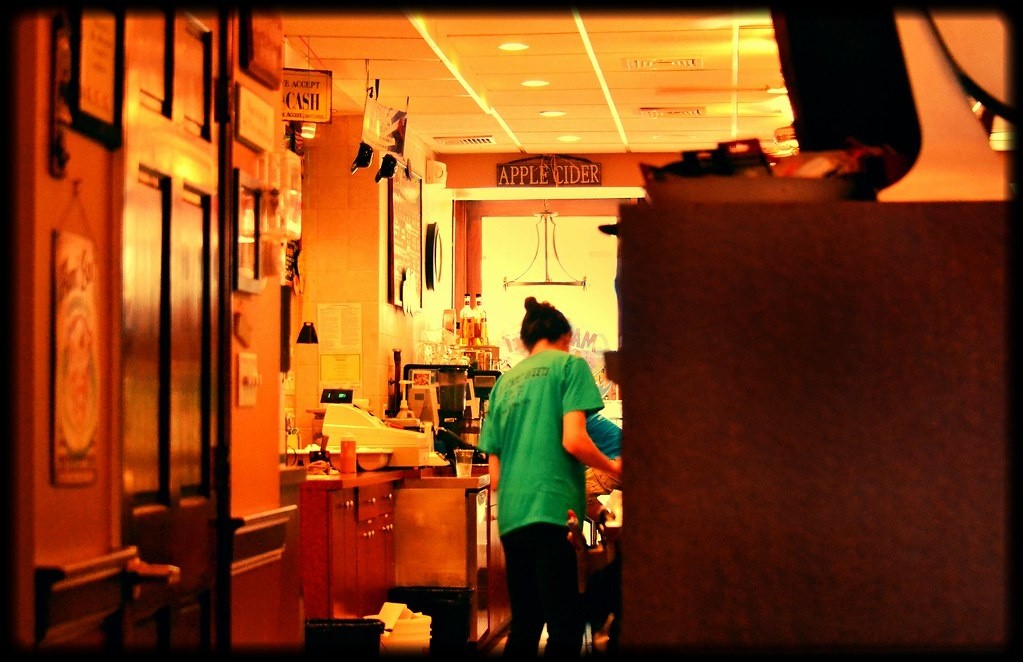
[
  {"left": 472, "top": 293, "right": 489, "bottom": 347},
  {"left": 340, "top": 431, "right": 356, "bottom": 473},
  {"left": 459, "top": 294, "right": 475, "bottom": 346},
  {"left": 442, "top": 308, "right": 457, "bottom": 335}
]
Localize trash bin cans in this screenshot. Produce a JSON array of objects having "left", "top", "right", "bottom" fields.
[{"left": 307, "top": 617, "right": 386, "bottom": 662}]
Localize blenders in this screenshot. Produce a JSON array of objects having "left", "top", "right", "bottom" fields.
[{"left": 437, "top": 366, "right": 468, "bottom": 458}]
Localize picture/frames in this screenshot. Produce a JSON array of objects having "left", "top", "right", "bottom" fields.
[
  {"left": 51, "top": 13, "right": 126, "bottom": 151},
  {"left": 424, "top": 221, "right": 443, "bottom": 293}
]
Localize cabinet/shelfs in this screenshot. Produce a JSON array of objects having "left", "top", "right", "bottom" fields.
[{"left": 301, "top": 482, "right": 394, "bottom": 618}]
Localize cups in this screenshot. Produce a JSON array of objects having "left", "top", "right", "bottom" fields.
[{"left": 454, "top": 450, "right": 474, "bottom": 478}]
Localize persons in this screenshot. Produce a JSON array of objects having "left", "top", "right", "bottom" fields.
[
  {"left": 477, "top": 296, "right": 621, "bottom": 662},
  {"left": 584, "top": 413, "right": 622, "bottom": 521}
]
